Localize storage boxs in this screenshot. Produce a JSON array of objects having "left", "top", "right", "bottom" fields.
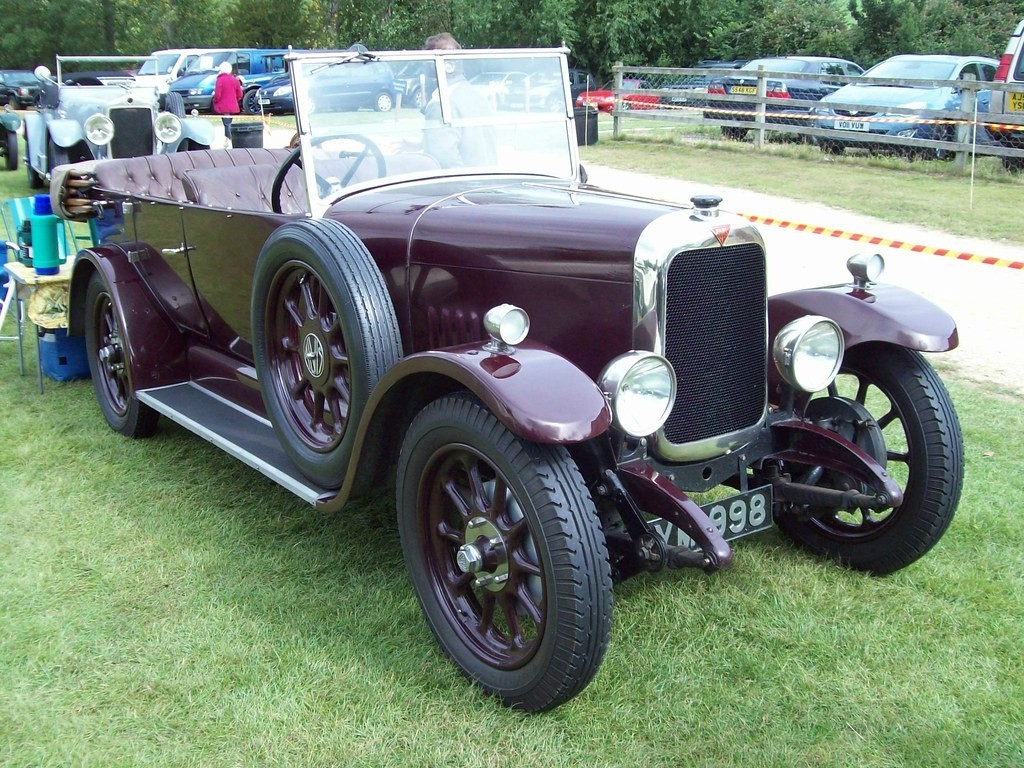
[{"left": 38, "top": 327, "right": 91, "bottom": 383}]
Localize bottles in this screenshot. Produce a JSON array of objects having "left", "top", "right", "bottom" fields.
[{"left": 17, "top": 221, "right": 31, "bottom": 266}]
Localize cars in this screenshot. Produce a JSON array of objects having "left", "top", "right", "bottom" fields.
[
  {"left": 255, "top": 53, "right": 397, "bottom": 112},
  {"left": 809, "top": 54, "right": 999, "bottom": 162},
  {"left": 133, "top": 48, "right": 234, "bottom": 109},
  {"left": 576, "top": 79, "right": 660, "bottom": 117},
  {"left": 467, "top": 72, "right": 529, "bottom": 110},
  {"left": 0, "top": 70, "right": 43, "bottom": 110},
  {"left": 528, "top": 70, "right": 597, "bottom": 113},
  {"left": 703, "top": 56, "right": 865, "bottom": 140},
  {"left": 22, "top": 52, "right": 215, "bottom": 189},
  {"left": 661, "top": 61, "right": 745, "bottom": 107},
  {"left": 0, "top": 112, "right": 21, "bottom": 170},
  {"left": 49, "top": 46, "right": 966, "bottom": 713},
  {"left": 393, "top": 60, "right": 436, "bottom": 108}
]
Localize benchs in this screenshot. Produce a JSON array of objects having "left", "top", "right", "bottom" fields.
[
  {"left": 94, "top": 146, "right": 329, "bottom": 203},
  {"left": 182, "top": 152, "right": 444, "bottom": 215}
]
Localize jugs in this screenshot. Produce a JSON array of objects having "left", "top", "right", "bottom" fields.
[{"left": 31, "top": 194, "right": 67, "bottom": 276}]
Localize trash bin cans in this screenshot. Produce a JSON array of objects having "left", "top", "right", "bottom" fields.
[
  {"left": 573, "top": 107, "right": 598, "bottom": 146},
  {"left": 230, "top": 122, "right": 265, "bottom": 149}
]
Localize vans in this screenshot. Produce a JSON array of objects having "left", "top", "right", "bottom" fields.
[
  {"left": 985, "top": 18, "right": 1024, "bottom": 173},
  {"left": 169, "top": 50, "right": 309, "bottom": 115}
]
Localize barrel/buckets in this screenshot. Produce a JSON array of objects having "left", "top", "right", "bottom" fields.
[
  {"left": 230, "top": 121, "right": 264, "bottom": 149},
  {"left": 574, "top": 107, "right": 598, "bottom": 145}
]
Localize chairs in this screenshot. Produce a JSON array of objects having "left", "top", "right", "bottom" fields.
[
  {"left": 0, "top": 241, "right": 27, "bottom": 340},
  {"left": 0, "top": 194, "right": 92, "bottom": 263}
]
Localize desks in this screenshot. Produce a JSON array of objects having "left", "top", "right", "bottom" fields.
[{"left": 3, "top": 257, "right": 78, "bottom": 395}]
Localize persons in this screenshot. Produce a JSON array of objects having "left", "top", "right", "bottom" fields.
[
  {"left": 212, "top": 62, "right": 243, "bottom": 115},
  {"left": 421, "top": 33, "right": 485, "bottom": 167}
]
